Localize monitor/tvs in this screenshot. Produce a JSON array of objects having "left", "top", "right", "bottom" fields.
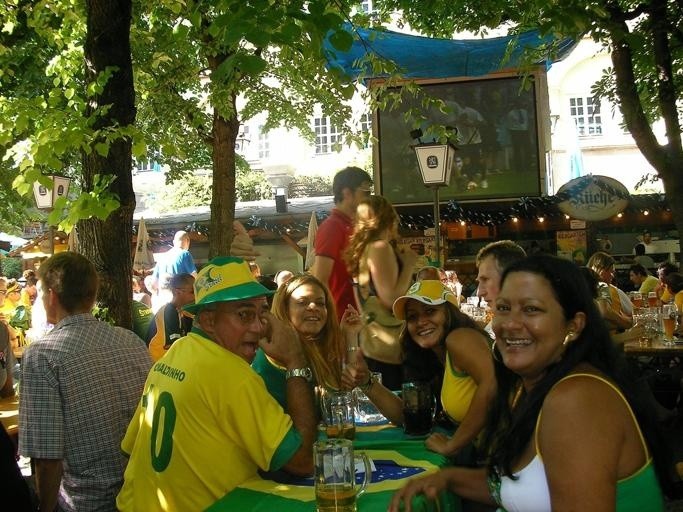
[{"left": 366, "top": 62, "right": 547, "bottom": 208}]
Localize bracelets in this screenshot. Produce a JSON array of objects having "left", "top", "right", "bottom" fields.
[{"left": 358, "top": 370, "right": 375, "bottom": 396}]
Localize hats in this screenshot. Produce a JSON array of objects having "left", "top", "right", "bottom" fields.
[
  {"left": 392, "top": 279, "right": 461, "bottom": 321},
  {"left": 180, "top": 255, "right": 279, "bottom": 311}
]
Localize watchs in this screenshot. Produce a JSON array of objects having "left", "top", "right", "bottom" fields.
[
  {"left": 345, "top": 347, "right": 361, "bottom": 352},
  {"left": 285, "top": 367, "right": 313, "bottom": 383}
]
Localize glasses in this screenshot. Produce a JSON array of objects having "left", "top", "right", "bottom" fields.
[
  {"left": 213, "top": 305, "right": 272, "bottom": 326},
  {"left": 352, "top": 188, "right": 375, "bottom": 194}
]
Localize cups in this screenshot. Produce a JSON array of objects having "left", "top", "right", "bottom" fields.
[
  {"left": 467, "top": 296, "right": 480, "bottom": 306},
  {"left": 661, "top": 304, "right": 675, "bottom": 344},
  {"left": 401, "top": 381, "right": 439, "bottom": 440},
  {"left": 472, "top": 306, "right": 485, "bottom": 320},
  {"left": 320, "top": 390, "right": 356, "bottom": 440},
  {"left": 461, "top": 303, "right": 474, "bottom": 314},
  {"left": 633, "top": 291, "right": 642, "bottom": 308},
  {"left": 636, "top": 315, "right": 653, "bottom": 347},
  {"left": 310, "top": 437, "right": 373, "bottom": 512},
  {"left": 647, "top": 292, "right": 657, "bottom": 312}
]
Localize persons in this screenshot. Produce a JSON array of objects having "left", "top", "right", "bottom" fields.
[
  {"left": 341, "top": 280, "right": 509, "bottom": 464},
  {"left": 342, "top": 195, "right": 419, "bottom": 391},
  {"left": 629, "top": 264, "right": 663, "bottom": 298},
  {"left": 527, "top": 241, "right": 540, "bottom": 254},
  {"left": 585, "top": 253, "right": 632, "bottom": 330},
  {"left": 476, "top": 241, "right": 527, "bottom": 341},
  {"left": 389, "top": 253, "right": 682, "bottom": 511},
  {"left": 445, "top": 270, "right": 464, "bottom": 309},
  {"left": 26, "top": 256, "right": 54, "bottom": 339},
  {"left": 151, "top": 230, "right": 197, "bottom": 313},
  {"left": 249, "top": 262, "right": 260, "bottom": 280},
  {"left": 606, "top": 262, "right": 642, "bottom": 345},
  {"left": 416, "top": 266, "right": 441, "bottom": 280},
  {"left": 5, "top": 279, "right": 26, "bottom": 316},
  {"left": 17, "top": 269, "right": 36, "bottom": 287},
  {"left": 633, "top": 244, "right": 656, "bottom": 271},
  {"left": 17, "top": 278, "right": 37, "bottom": 312},
  {"left": 0, "top": 278, "right": 16, "bottom": 340},
  {"left": 115, "top": 255, "right": 317, "bottom": 512},
  {"left": 251, "top": 275, "right": 364, "bottom": 417},
  {"left": 0, "top": 321, "right": 36, "bottom": 512},
  {"left": 653, "top": 261, "right": 679, "bottom": 300},
  {"left": 16, "top": 251, "right": 152, "bottom": 512},
  {"left": 572, "top": 250, "right": 586, "bottom": 267},
  {"left": 633, "top": 229, "right": 659, "bottom": 254},
  {"left": 311, "top": 166, "right": 374, "bottom": 325},
  {"left": 145, "top": 272, "right": 195, "bottom": 363},
  {"left": 666, "top": 272, "right": 683, "bottom": 314}
]
